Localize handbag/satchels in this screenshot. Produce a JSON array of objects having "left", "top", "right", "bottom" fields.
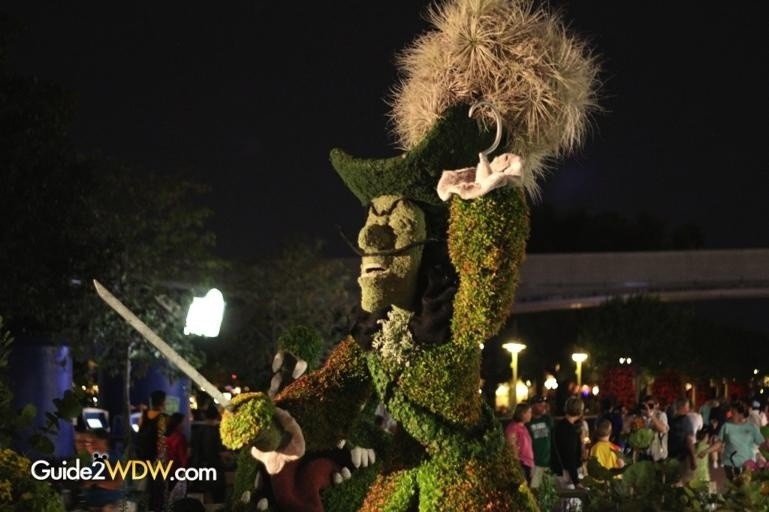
[{"left": 629, "top": 428, "right": 655, "bottom": 449}]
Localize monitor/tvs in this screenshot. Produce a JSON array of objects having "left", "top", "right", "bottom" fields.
[
  {"left": 128, "top": 412, "right": 143, "bottom": 433},
  {"left": 81, "top": 407, "right": 111, "bottom": 432}
]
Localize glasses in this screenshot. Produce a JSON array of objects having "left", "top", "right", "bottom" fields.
[{"left": 640, "top": 404, "right": 656, "bottom": 410}]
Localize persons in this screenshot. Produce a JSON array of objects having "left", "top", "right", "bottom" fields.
[
  {"left": 130, "top": 390, "right": 227, "bottom": 512},
  {"left": 505, "top": 394, "right": 769, "bottom": 512}
]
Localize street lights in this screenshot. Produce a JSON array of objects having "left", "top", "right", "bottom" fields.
[
  {"left": 572, "top": 350, "right": 588, "bottom": 386},
  {"left": 501, "top": 341, "right": 526, "bottom": 410}
]
